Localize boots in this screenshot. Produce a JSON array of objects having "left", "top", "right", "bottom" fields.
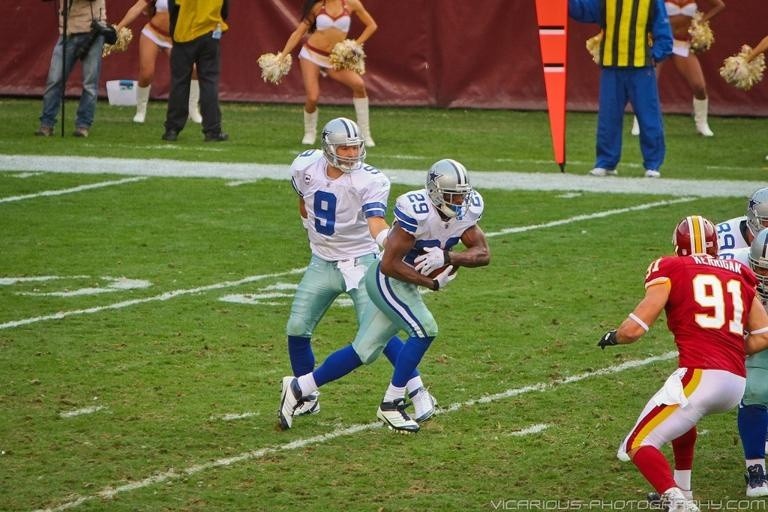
[
  {"left": 692, "top": 96, "right": 714, "bottom": 137},
  {"left": 352, "top": 97, "right": 376, "bottom": 147},
  {"left": 189, "top": 79, "right": 203, "bottom": 123},
  {"left": 302, "top": 107, "right": 319, "bottom": 146},
  {"left": 132, "top": 85, "right": 152, "bottom": 123}
]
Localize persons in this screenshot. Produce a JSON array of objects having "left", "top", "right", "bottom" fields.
[
  {"left": 742, "top": 36, "right": 768, "bottom": 64},
  {"left": 630, "top": 0, "right": 726, "bottom": 138},
  {"left": 567, "top": 0, "right": 672, "bottom": 178},
  {"left": 109, "top": 1, "right": 204, "bottom": 124},
  {"left": 286, "top": 118, "right": 438, "bottom": 421},
  {"left": 161, "top": 1, "right": 228, "bottom": 141},
  {"left": 718, "top": 227, "right": 768, "bottom": 497},
  {"left": 278, "top": 158, "right": 490, "bottom": 436},
  {"left": 35, "top": 1, "right": 106, "bottom": 137},
  {"left": 276, "top": 0, "right": 378, "bottom": 148},
  {"left": 717, "top": 188, "right": 768, "bottom": 251},
  {"left": 598, "top": 216, "right": 768, "bottom": 511}
]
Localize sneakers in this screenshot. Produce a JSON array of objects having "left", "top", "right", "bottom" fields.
[
  {"left": 745, "top": 467, "right": 768, "bottom": 497},
  {"left": 73, "top": 127, "right": 88, "bottom": 138},
  {"left": 295, "top": 396, "right": 320, "bottom": 416},
  {"left": 376, "top": 400, "right": 420, "bottom": 434},
  {"left": 631, "top": 115, "right": 640, "bottom": 136},
  {"left": 36, "top": 125, "right": 56, "bottom": 138},
  {"left": 590, "top": 167, "right": 617, "bottom": 176},
  {"left": 204, "top": 132, "right": 228, "bottom": 142},
  {"left": 645, "top": 169, "right": 661, "bottom": 179},
  {"left": 161, "top": 129, "right": 179, "bottom": 140},
  {"left": 278, "top": 376, "right": 304, "bottom": 429},
  {"left": 413, "top": 390, "right": 437, "bottom": 422},
  {"left": 648, "top": 489, "right": 694, "bottom": 501},
  {"left": 664, "top": 502, "right": 698, "bottom": 512}
]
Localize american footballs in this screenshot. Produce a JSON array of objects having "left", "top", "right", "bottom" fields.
[{"left": 416, "top": 258, "right": 459, "bottom": 278}]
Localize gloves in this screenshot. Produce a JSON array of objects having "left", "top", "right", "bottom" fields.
[
  {"left": 597, "top": 329, "right": 617, "bottom": 350},
  {"left": 430, "top": 264, "right": 458, "bottom": 293},
  {"left": 414, "top": 245, "right": 448, "bottom": 276}
]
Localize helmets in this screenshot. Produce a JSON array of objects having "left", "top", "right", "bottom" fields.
[
  {"left": 744, "top": 187, "right": 768, "bottom": 241},
  {"left": 322, "top": 117, "right": 365, "bottom": 175},
  {"left": 426, "top": 158, "right": 471, "bottom": 219},
  {"left": 750, "top": 227, "right": 767, "bottom": 306},
  {"left": 673, "top": 215, "right": 718, "bottom": 256}
]
